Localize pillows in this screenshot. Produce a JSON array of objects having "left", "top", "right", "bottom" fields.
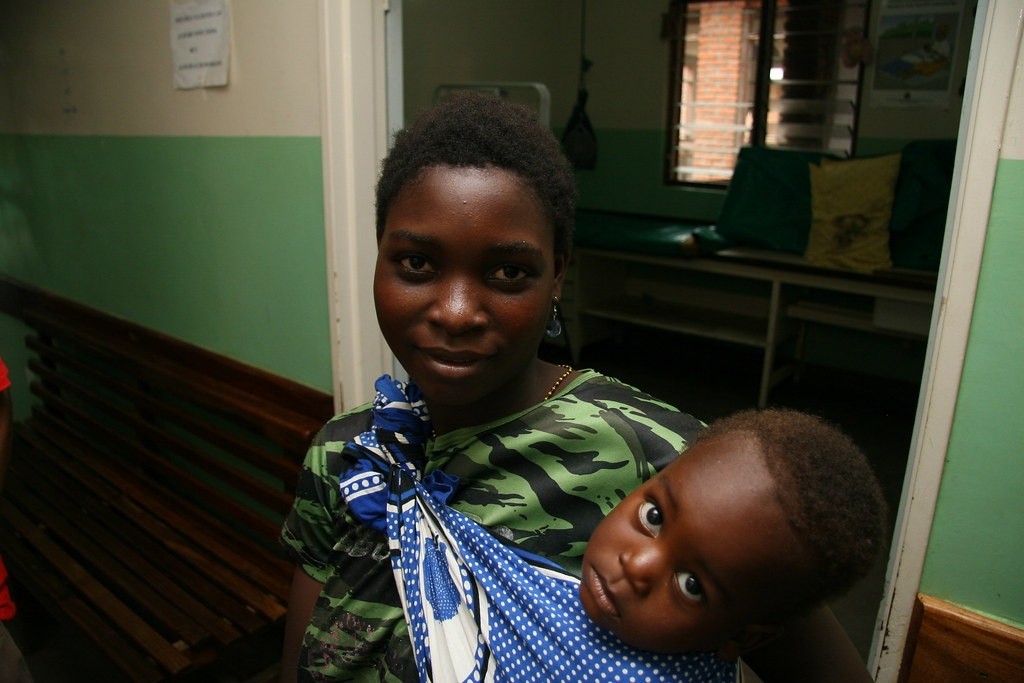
[
  {"left": 805, "top": 155, "right": 902, "bottom": 271},
  {"left": 888, "top": 138, "right": 957, "bottom": 270},
  {"left": 716, "top": 146, "right": 843, "bottom": 256}
]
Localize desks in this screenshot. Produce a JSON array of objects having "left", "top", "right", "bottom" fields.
[{"left": 564, "top": 207, "right": 938, "bottom": 412}]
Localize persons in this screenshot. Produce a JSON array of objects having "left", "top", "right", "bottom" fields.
[
  {"left": 0, "top": 358, "right": 16, "bottom": 623},
  {"left": 264, "top": 86, "right": 886, "bottom": 683}
]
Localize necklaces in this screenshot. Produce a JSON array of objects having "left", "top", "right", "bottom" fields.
[{"left": 428, "top": 365, "right": 572, "bottom": 437}]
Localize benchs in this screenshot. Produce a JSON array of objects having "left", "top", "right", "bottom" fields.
[{"left": 0, "top": 290, "right": 334, "bottom": 682}]
